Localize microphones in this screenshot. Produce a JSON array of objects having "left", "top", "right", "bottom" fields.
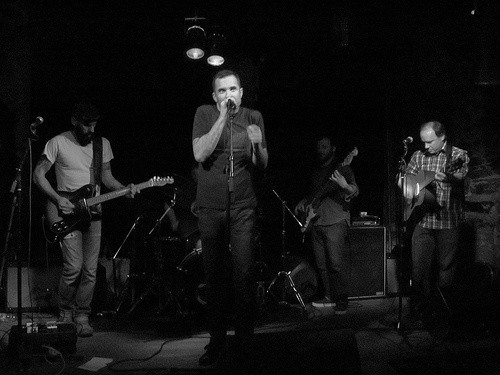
[
  {"left": 227, "top": 97, "right": 237, "bottom": 111},
  {"left": 404, "top": 136, "right": 413, "bottom": 143},
  {"left": 30, "top": 116, "right": 44, "bottom": 128}
]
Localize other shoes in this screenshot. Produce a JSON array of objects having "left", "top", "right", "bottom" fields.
[
  {"left": 74, "top": 316, "right": 94, "bottom": 336},
  {"left": 200, "top": 338, "right": 226, "bottom": 365},
  {"left": 59, "top": 315, "right": 72, "bottom": 323},
  {"left": 312, "top": 298, "right": 348, "bottom": 314}
]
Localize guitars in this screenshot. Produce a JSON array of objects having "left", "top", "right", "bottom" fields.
[
  {"left": 404, "top": 152, "right": 470, "bottom": 223},
  {"left": 42, "top": 176, "right": 174, "bottom": 238},
  {"left": 295, "top": 147, "right": 358, "bottom": 235}
]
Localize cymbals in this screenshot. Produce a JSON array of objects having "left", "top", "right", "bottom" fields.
[{"left": 157, "top": 174, "right": 197, "bottom": 209}]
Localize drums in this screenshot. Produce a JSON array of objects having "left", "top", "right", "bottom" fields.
[{"left": 177, "top": 229, "right": 207, "bottom": 319}]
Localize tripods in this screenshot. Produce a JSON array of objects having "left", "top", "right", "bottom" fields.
[
  {"left": 263, "top": 189, "right": 308, "bottom": 309},
  {"left": 111, "top": 190, "right": 177, "bottom": 326}
]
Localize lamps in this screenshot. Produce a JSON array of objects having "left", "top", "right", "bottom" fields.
[
  {"left": 205, "top": 24, "right": 226, "bottom": 66},
  {"left": 183, "top": 16, "right": 208, "bottom": 62}
]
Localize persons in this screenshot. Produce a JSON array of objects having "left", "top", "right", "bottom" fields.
[
  {"left": 32, "top": 100, "right": 141, "bottom": 337},
  {"left": 402, "top": 121, "right": 471, "bottom": 329},
  {"left": 192, "top": 70, "right": 269, "bottom": 365},
  {"left": 295, "top": 138, "right": 360, "bottom": 315}
]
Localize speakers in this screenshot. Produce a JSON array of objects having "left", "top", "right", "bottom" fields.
[
  {"left": 421, "top": 271, "right": 500, "bottom": 341},
  {"left": 341, "top": 223, "right": 387, "bottom": 299}
]
